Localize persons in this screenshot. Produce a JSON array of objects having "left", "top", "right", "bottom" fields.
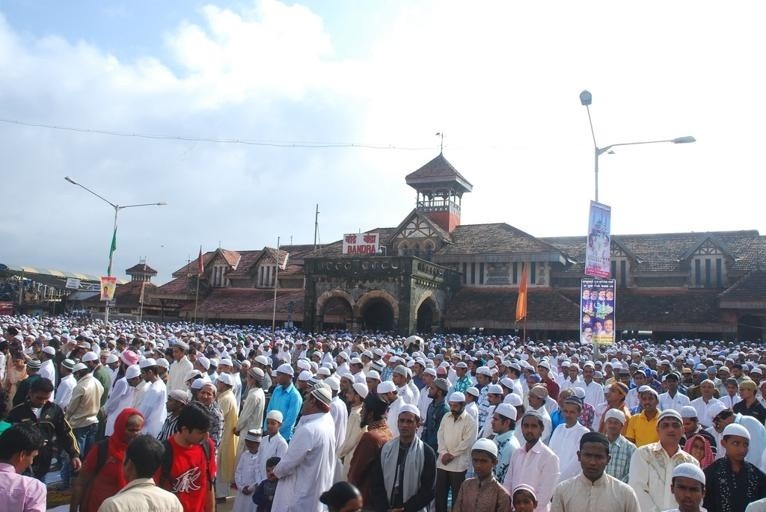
[{"left": 0, "top": 312, "right": 766, "bottom": 511}]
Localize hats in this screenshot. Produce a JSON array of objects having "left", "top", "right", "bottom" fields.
[
  {"left": 191, "top": 378, "right": 206, "bottom": 389},
  {"left": 217, "top": 342, "right": 224, "bottom": 348},
  {"left": 407, "top": 360, "right": 415, "bottom": 367},
  {"left": 574, "top": 388, "right": 585, "bottom": 398},
  {"left": 109, "top": 340, "right": 116, "bottom": 347},
  {"left": 247, "top": 367, "right": 264, "bottom": 382},
  {"left": 350, "top": 358, "right": 361, "bottom": 365},
  {"left": 504, "top": 393, "right": 522, "bottom": 406},
  {"left": 571, "top": 362, "right": 579, "bottom": 369},
  {"left": 27, "top": 358, "right": 42, "bottom": 369},
  {"left": 343, "top": 373, "right": 355, "bottom": 382},
  {"left": 183, "top": 369, "right": 200, "bottom": 382},
  {"left": 55, "top": 328, "right": 63, "bottom": 334},
  {"left": 106, "top": 354, "right": 118, "bottom": 363},
  {"left": 226, "top": 343, "right": 233, "bottom": 348},
  {"left": 173, "top": 341, "right": 187, "bottom": 350},
  {"left": 740, "top": 380, "right": 757, "bottom": 390},
  {"left": 297, "top": 359, "right": 311, "bottom": 369},
  {"left": 707, "top": 366, "right": 717, "bottom": 373},
  {"left": 472, "top": 438, "right": 498, "bottom": 457},
  {"left": 604, "top": 408, "right": 626, "bottom": 426},
  {"left": 526, "top": 366, "right": 535, "bottom": 373},
  {"left": 614, "top": 382, "right": 628, "bottom": 396},
  {"left": 496, "top": 403, "right": 518, "bottom": 421},
  {"left": 314, "top": 383, "right": 329, "bottom": 389},
  {"left": 276, "top": 340, "right": 282, "bottom": 344},
  {"left": 513, "top": 484, "right": 537, "bottom": 501},
  {"left": 82, "top": 352, "right": 98, "bottom": 362},
  {"left": 456, "top": 362, "right": 467, "bottom": 368},
  {"left": 539, "top": 361, "right": 549, "bottom": 368},
  {"left": 710, "top": 404, "right": 727, "bottom": 422},
  {"left": 253, "top": 340, "right": 259, "bottom": 346},
  {"left": 70, "top": 340, "right": 77, "bottom": 344},
  {"left": 500, "top": 378, "right": 514, "bottom": 389},
  {"left": 612, "top": 362, "right": 622, "bottom": 368},
  {"left": 266, "top": 410, "right": 283, "bottom": 423},
  {"left": 523, "top": 410, "right": 542, "bottom": 422},
  {"left": 532, "top": 374, "right": 541, "bottom": 381},
  {"left": 324, "top": 377, "right": 340, "bottom": 390},
  {"left": 561, "top": 361, "right": 570, "bottom": 368},
  {"left": 394, "top": 367, "right": 408, "bottom": 378},
  {"left": 377, "top": 381, "right": 397, "bottom": 393},
  {"left": 389, "top": 356, "right": 399, "bottom": 363},
  {"left": 751, "top": 368, "right": 762, "bottom": 374},
  {"left": 639, "top": 385, "right": 651, "bottom": 392},
  {"left": 436, "top": 354, "right": 444, "bottom": 361},
  {"left": 518, "top": 360, "right": 530, "bottom": 367},
  {"left": 595, "top": 360, "right": 602, "bottom": 365},
  {"left": 198, "top": 357, "right": 209, "bottom": 370},
  {"left": 657, "top": 409, "right": 684, "bottom": 425},
  {"left": 424, "top": 368, "right": 437, "bottom": 376},
  {"left": 242, "top": 360, "right": 251, "bottom": 367},
  {"left": 488, "top": 384, "right": 503, "bottom": 393},
  {"left": 723, "top": 423, "right": 750, "bottom": 439},
  {"left": 565, "top": 396, "right": 583, "bottom": 411},
  {"left": 449, "top": 392, "right": 466, "bottom": 402},
  {"left": 210, "top": 358, "right": 219, "bottom": 365},
  {"left": 583, "top": 360, "right": 594, "bottom": 369},
  {"left": 313, "top": 351, "right": 321, "bottom": 357},
  {"left": 139, "top": 358, "right": 156, "bottom": 369},
  {"left": 427, "top": 352, "right": 435, "bottom": 359},
  {"left": 126, "top": 366, "right": 141, "bottom": 379},
  {"left": 221, "top": 359, "right": 233, "bottom": 365},
  {"left": 666, "top": 372, "right": 681, "bottom": 380},
  {"left": 374, "top": 349, "right": 382, "bottom": 356},
  {"left": 100, "top": 351, "right": 109, "bottom": 357},
  {"left": 311, "top": 388, "right": 333, "bottom": 407},
  {"left": 352, "top": 383, "right": 368, "bottom": 399},
  {"left": 466, "top": 387, "right": 479, "bottom": 396},
  {"left": 621, "top": 369, "right": 630, "bottom": 373},
  {"left": 53, "top": 335, "right": 61, "bottom": 340},
  {"left": 61, "top": 333, "right": 69, "bottom": 338},
  {"left": 416, "top": 360, "right": 426, "bottom": 367},
  {"left": 509, "top": 363, "right": 522, "bottom": 370},
  {"left": 93, "top": 338, "right": 100, "bottom": 343},
  {"left": 682, "top": 368, "right": 691, "bottom": 373},
  {"left": 531, "top": 386, "right": 548, "bottom": 400},
  {"left": 476, "top": 366, "right": 494, "bottom": 377},
  {"left": 263, "top": 342, "right": 269, "bottom": 346},
  {"left": 434, "top": 377, "right": 450, "bottom": 391},
  {"left": 27, "top": 335, "right": 36, "bottom": 340},
  {"left": 696, "top": 364, "right": 707, "bottom": 370},
  {"left": 399, "top": 404, "right": 421, "bottom": 416},
  {"left": 41, "top": 346, "right": 55, "bottom": 355},
  {"left": 319, "top": 367, "right": 331, "bottom": 374},
  {"left": 217, "top": 374, "right": 232, "bottom": 386},
  {"left": 245, "top": 429, "right": 262, "bottom": 442},
  {"left": 363, "top": 350, "right": 373, "bottom": 359},
  {"left": 365, "top": 371, "right": 380, "bottom": 378},
  {"left": 295, "top": 340, "right": 302, "bottom": 344},
  {"left": 488, "top": 360, "right": 497, "bottom": 368},
  {"left": 718, "top": 366, "right": 729, "bottom": 372},
  {"left": 61, "top": 358, "right": 75, "bottom": 370},
  {"left": 72, "top": 363, "right": 87, "bottom": 373},
  {"left": 371, "top": 361, "right": 383, "bottom": 372},
  {"left": 254, "top": 355, "right": 268, "bottom": 365},
  {"left": 79, "top": 342, "right": 90, "bottom": 349},
  {"left": 298, "top": 371, "right": 313, "bottom": 380},
  {"left": 593, "top": 371, "right": 603, "bottom": 378},
  {"left": 276, "top": 364, "right": 294, "bottom": 376},
  {"left": 71, "top": 327, "right": 79, "bottom": 332},
  {"left": 157, "top": 358, "right": 169, "bottom": 370},
  {"left": 437, "top": 366, "right": 447, "bottom": 376},
  {"left": 323, "top": 362, "right": 333, "bottom": 368},
  {"left": 311, "top": 361, "right": 318, "bottom": 372},
  {"left": 725, "top": 378, "right": 737, "bottom": 385},
  {"left": 339, "top": 351, "right": 348, "bottom": 359},
  {"left": 672, "top": 463, "right": 706, "bottom": 484},
  {"left": 168, "top": 390, "right": 187, "bottom": 406},
  {"left": 121, "top": 350, "right": 139, "bottom": 365},
  {"left": 680, "top": 406, "right": 697, "bottom": 417}
]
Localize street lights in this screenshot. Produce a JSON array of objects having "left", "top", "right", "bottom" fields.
[
  {"left": 64, "top": 175, "right": 168, "bottom": 328},
  {"left": 579, "top": 86, "right": 697, "bottom": 361}
]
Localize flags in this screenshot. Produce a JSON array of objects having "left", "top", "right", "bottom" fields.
[
  {"left": 198, "top": 246, "right": 204, "bottom": 277},
  {"left": 515, "top": 257, "right": 527, "bottom": 321},
  {"left": 107, "top": 226, "right": 116, "bottom": 276}
]
